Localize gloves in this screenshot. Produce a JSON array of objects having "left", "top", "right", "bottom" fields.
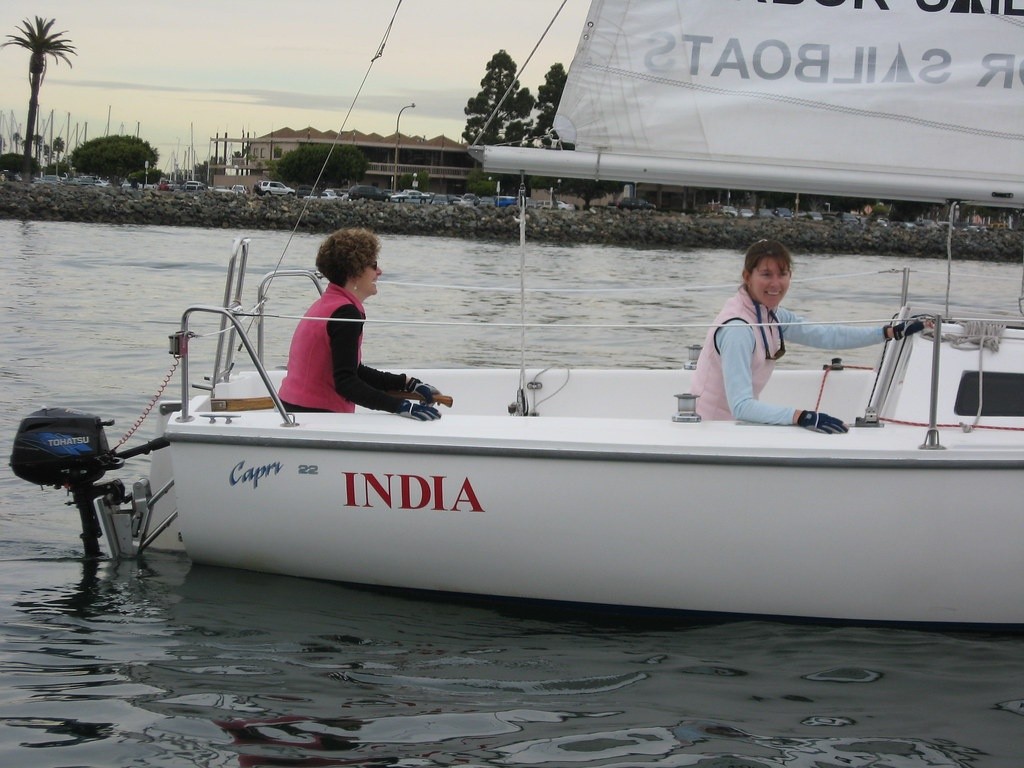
[
  {"left": 406, "top": 377, "right": 441, "bottom": 407},
  {"left": 892, "top": 314, "right": 936, "bottom": 340},
  {"left": 398, "top": 399, "right": 441, "bottom": 421},
  {"left": 797, "top": 409, "right": 849, "bottom": 435}
]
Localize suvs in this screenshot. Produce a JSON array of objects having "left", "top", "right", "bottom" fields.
[{"left": 259, "top": 180, "right": 296, "bottom": 198}]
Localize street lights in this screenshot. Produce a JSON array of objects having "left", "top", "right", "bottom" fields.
[{"left": 393, "top": 104, "right": 416, "bottom": 192}]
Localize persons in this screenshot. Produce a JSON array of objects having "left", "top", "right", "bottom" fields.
[
  {"left": 275, "top": 226, "right": 444, "bottom": 425},
  {"left": 691, "top": 240, "right": 934, "bottom": 435}
]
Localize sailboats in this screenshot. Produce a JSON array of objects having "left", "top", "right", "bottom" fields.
[{"left": 9, "top": 1, "right": 1024, "bottom": 626}]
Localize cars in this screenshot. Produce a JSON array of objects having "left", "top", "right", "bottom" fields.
[
  {"left": 299, "top": 184, "right": 576, "bottom": 214},
  {"left": 717, "top": 202, "right": 824, "bottom": 225},
  {"left": 42, "top": 174, "right": 63, "bottom": 184},
  {"left": 79, "top": 174, "right": 249, "bottom": 198},
  {"left": 615, "top": 198, "right": 656, "bottom": 212}
]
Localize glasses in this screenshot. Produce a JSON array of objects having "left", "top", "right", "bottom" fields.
[
  {"left": 370, "top": 260, "right": 377, "bottom": 270},
  {"left": 764, "top": 340, "right": 786, "bottom": 361}
]
